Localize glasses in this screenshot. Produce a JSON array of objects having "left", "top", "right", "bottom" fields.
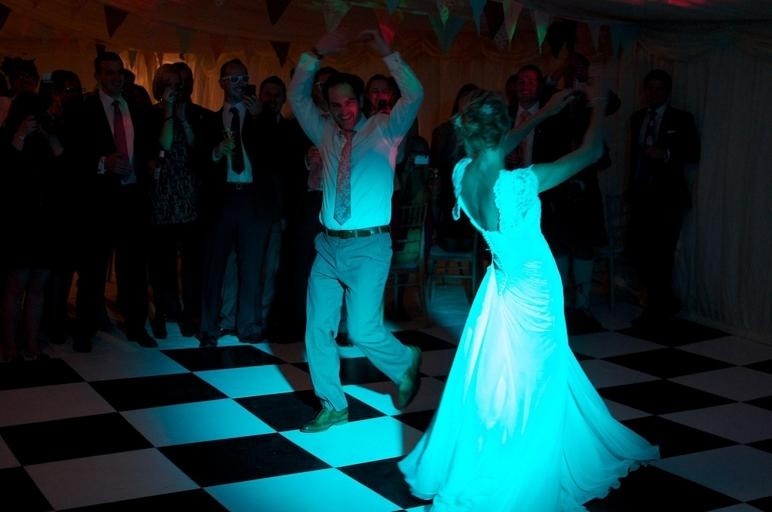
[{"left": 220, "top": 73, "right": 249, "bottom": 86}]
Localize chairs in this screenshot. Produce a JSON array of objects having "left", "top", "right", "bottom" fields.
[
  {"left": 383, "top": 201, "right": 427, "bottom": 315},
  {"left": 429, "top": 205, "right": 478, "bottom": 304},
  {"left": 599, "top": 190, "right": 650, "bottom": 320}
]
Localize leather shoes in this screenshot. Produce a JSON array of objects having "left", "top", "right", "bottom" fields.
[
  {"left": 298, "top": 405, "right": 350, "bottom": 434},
  {"left": 395, "top": 345, "right": 423, "bottom": 412},
  {"left": 73, "top": 311, "right": 264, "bottom": 354}
]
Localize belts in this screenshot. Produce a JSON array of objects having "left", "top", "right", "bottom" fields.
[{"left": 321, "top": 223, "right": 391, "bottom": 240}]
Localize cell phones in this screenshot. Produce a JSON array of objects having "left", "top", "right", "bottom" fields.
[{"left": 244, "top": 84, "right": 257, "bottom": 97}]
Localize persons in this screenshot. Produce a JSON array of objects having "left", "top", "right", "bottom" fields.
[
  {"left": 445, "top": 87, "right": 620, "bottom": 511},
  {"left": 286, "top": 27, "right": 424, "bottom": 431}
]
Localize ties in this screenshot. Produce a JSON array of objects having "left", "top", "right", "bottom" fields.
[
  {"left": 642, "top": 111, "right": 655, "bottom": 151},
  {"left": 333, "top": 128, "right": 355, "bottom": 225},
  {"left": 515, "top": 111, "right": 529, "bottom": 158},
  {"left": 110, "top": 99, "right": 132, "bottom": 183},
  {"left": 227, "top": 106, "right": 246, "bottom": 176}
]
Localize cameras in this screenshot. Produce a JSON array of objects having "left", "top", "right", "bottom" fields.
[{"left": 32, "top": 112, "right": 53, "bottom": 130}]
